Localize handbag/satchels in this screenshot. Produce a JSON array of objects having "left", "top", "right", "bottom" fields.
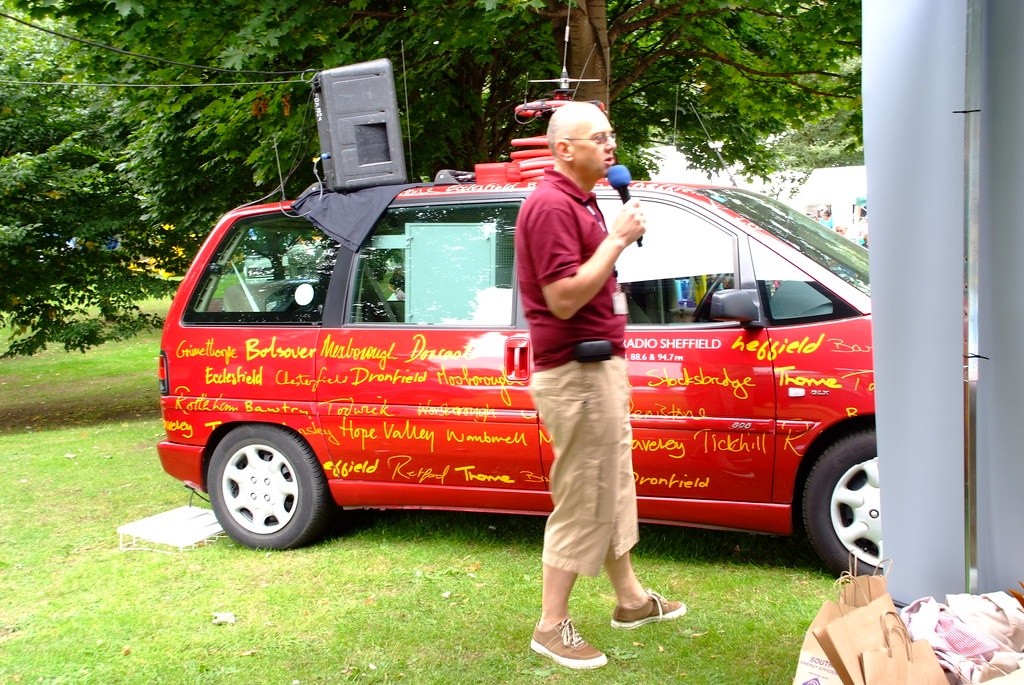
[{"left": 792, "top": 547, "right": 950, "bottom": 685}]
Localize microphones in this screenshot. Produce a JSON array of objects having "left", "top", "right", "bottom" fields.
[{"left": 608, "top": 165, "right": 643, "bottom": 247}]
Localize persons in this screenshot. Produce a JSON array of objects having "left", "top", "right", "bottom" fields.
[
  {"left": 513, "top": 102, "right": 687, "bottom": 669},
  {"left": 390, "top": 267, "right": 405, "bottom": 293},
  {"left": 820, "top": 209, "right": 869, "bottom": 249}
]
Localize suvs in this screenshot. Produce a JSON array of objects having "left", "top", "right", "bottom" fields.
[{"left": 157, "top": 180, "right": 980, "bottom": 581}]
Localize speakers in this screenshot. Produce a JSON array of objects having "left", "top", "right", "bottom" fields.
[{"left": 311, "top": 57, "right": 408, "bottom": 193}]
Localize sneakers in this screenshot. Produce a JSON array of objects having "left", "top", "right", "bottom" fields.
[
  {"left": 610, "top": 588, "right": 687, "bottom": 631},
  {"left": 530, "top": 619, "right": 608, "bottom": 669}
]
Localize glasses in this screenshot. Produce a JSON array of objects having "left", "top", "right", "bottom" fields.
[{"left": 561, "top": 132, "right": 617, "bottom": 145}]
